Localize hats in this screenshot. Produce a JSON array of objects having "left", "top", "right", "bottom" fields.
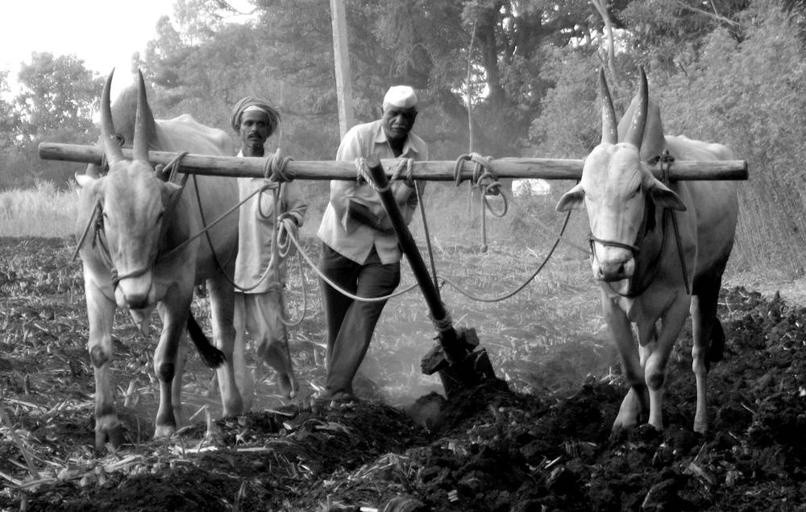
[
  {"left": 382, "top": 82, "right": 418, "bottom": 112},
  {"left": 231, "top": 96, "right": 278, "bottom": 138}
]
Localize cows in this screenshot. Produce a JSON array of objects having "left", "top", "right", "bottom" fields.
[
  {"left": 75, "top": 66, "right": 242, "bottom": 452},
  {"left": 556, "top": 64, "right": 739, "bottom": 437}
]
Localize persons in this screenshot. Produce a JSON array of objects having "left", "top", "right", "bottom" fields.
[
  {"left": 315, "top": 85, "right": 429, "bottom": 411},
  {"left": 229, "top": 99, "right": 303, "bottom": 418}
]
